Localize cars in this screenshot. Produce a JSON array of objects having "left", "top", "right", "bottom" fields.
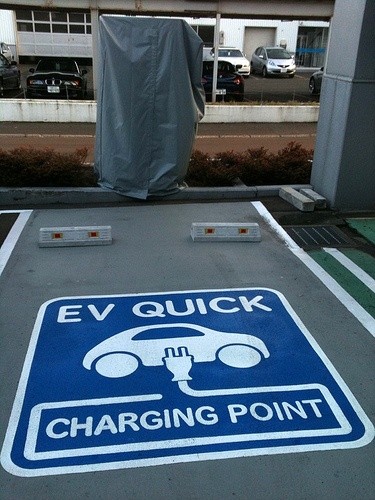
[
  {"left": 25, "top": 56, "right": 88, "bottom": 101},
  {"left": 251, "top": 46, "right": 297, "bottom": 78},
  {"left": 206, "top": 46, "right": 251, "bottom": 78},
  {"left": 200, "top": 61, "right": 245, "bottom": 103},
  {"left": 0, "top": 53, "right": 22, "bottom": 97}
]
